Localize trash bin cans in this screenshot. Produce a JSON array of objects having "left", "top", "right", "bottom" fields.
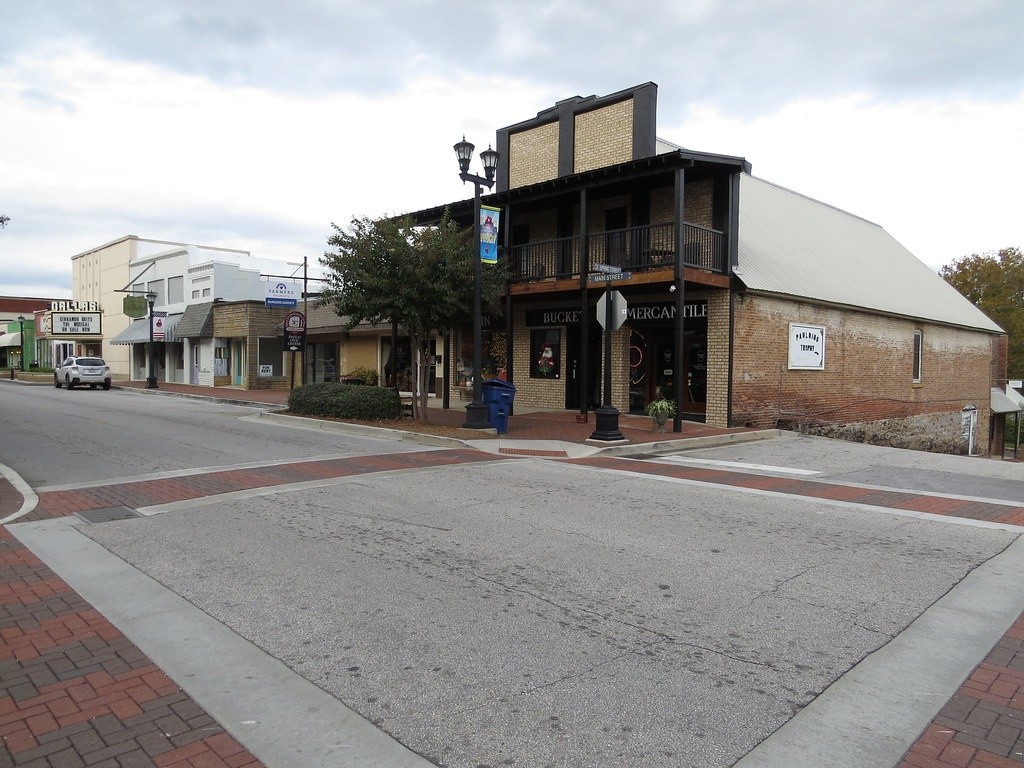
[{"left": 481, "top": 378, "right": 516, "bottom": 433}]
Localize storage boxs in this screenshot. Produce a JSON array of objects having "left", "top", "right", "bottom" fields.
[
  {"left": 482, "top": 378, "right": 516, "bottom": 404},
  {"left": 483, "top": 401, "right": 511, "bottom": 434}
]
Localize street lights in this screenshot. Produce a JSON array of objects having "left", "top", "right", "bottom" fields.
[
  {"left": 18, "top": 314, "right": 25, "bottom": 372},
  {"left": 146, "top": 290, "right": 159, "bottom": 388},
  {"left": 454, "top": 137, "right": 502, "bottom": 428}
]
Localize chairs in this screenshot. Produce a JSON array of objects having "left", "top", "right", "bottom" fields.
[
  {"left": 525, "top": 263, "right": 546, "bottom": 283},
  {"left": 619, "top": 240, "right": 701, "bottom": 272}
]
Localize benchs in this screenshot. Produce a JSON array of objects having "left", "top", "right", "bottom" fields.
[{"left": 400, "top": 395, "right": 419, "bottom": 419}]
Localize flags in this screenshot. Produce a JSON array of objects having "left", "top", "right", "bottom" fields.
[{"left": 479, "top": 203, "right": 500, "bottom": 264}]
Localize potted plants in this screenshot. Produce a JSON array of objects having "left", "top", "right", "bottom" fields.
[
  {"left": 340, "top": 366, "right": 380, "bottom": 387},
  {"left": 646, "top": 397, "right": 677, "bottom": 436}
]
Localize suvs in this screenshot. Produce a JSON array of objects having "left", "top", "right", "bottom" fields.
[{"left": 53, "top": 355, "right": 110, "bottom": 391}]
